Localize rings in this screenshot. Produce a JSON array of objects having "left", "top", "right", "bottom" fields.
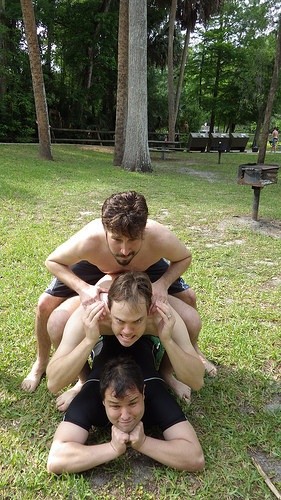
[{"left": 168, "top": 316, "right": 172, "bottom": 319}]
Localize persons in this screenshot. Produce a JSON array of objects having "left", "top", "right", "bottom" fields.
[
  {"left": 19, "top": 190, "right": 218, "bottom": 393},
  {"left": 271, "top": 127, "right": 279, "bottom": 153},
  {"left": 45, "top": 273, "right": 207, "bottom": 476}
]
